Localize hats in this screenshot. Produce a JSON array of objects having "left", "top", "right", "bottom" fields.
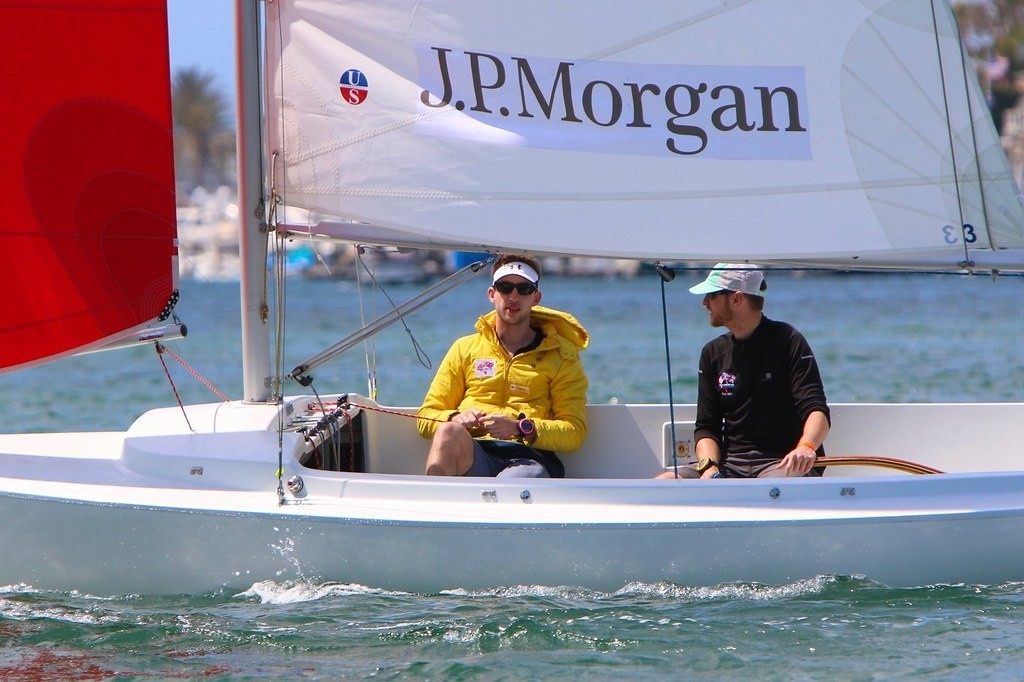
[
  {"left": 688, "top": 262, "right": 767, "bottom": 298},
  {"left": 492, "top": 262, "right": 538, "bottom": 286}
]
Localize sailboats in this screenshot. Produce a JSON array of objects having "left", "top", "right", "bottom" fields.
[{"left": 0, "top": 1, "right": 1024, "bottom": 595}]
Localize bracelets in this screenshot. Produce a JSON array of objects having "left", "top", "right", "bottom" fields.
[{"left": 797, "top": 441, "right": 815, "bottom": 450}]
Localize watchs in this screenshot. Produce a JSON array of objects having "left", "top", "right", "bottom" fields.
[
  {"left": 697, "top": 458, "right": 720, "bottom": 476},
  {"left": 518, "top": 413, "right": 535, "bottom": 437}
]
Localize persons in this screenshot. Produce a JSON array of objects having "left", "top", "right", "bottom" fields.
[
  {"left": 417, "top": 253, "right": 591, "bottom": 478},
  {"left": 654, "top": 263, "right": 831, "bottom": 479}
]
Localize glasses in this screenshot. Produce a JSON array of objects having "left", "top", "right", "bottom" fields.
[
  {"left": 495, "top": 280, "right": 534, "bottom": 295},
  {"left": 709, "top": 290, "right": 732, "bottom": 300}
]
